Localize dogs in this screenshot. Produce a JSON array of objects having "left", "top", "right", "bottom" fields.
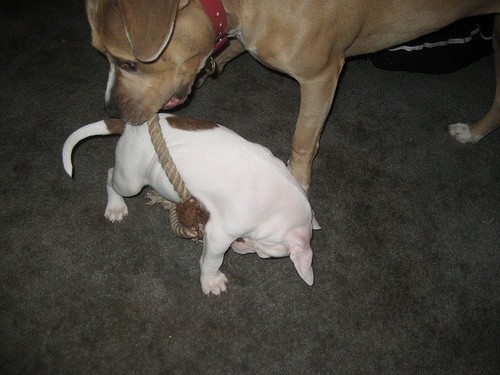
[
  {"left": 61, "top": 112, "right": 322, "bottom": 299},
  {"left": 87, "top": 0, "right": 500, "bottom": 193}
]
